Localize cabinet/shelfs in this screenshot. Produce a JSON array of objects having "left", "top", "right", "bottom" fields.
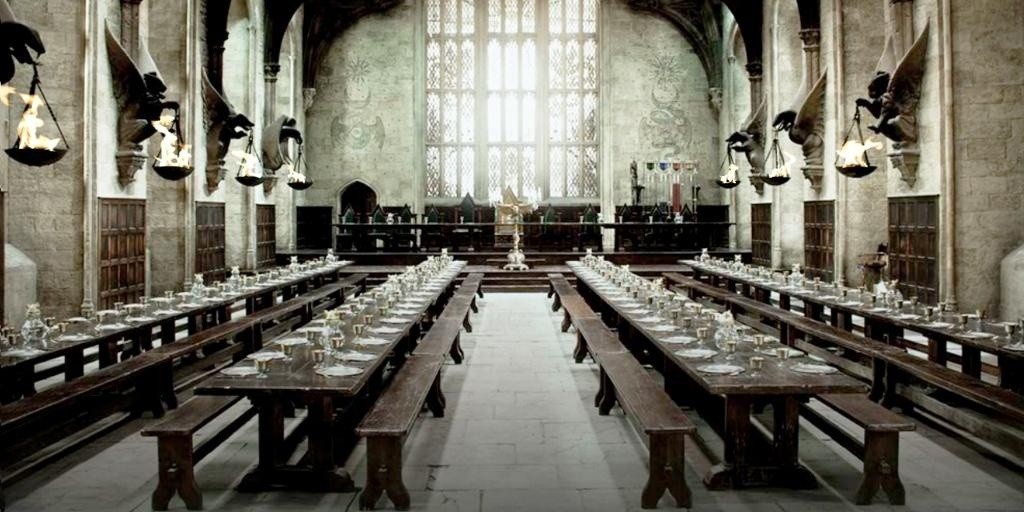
[
  {"left": 643, "top": 160, "right": 694, "bottom": 219},
  {"left": 190, "top": 272, "right": 207, "bottom": 299},
  {"left": 289, "top": 255, "right": 299, "bottom": 276},
  {"left": 326, "top": 248, "right": 335, "bottom": 267}
]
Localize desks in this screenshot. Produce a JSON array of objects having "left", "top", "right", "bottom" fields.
[
  {"left": 194, "top": 258, "right": 468, "bottom": 494},
  {"left": 567, "top": 260, "right": 864, "bottom": 491},
  {"left": 680, "top": 253, "right": 1024, "bottom": 395},
  {"left": 2, "top": 257, "right": 355, "bottom": 391}
]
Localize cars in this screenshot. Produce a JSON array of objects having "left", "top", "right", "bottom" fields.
[{"left": 2, "top": 282, "right": 220, "bottom": 350}]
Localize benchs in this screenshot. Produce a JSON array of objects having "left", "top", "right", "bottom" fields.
[
  {"left": 788, "top": 316, "right": 906, "bottom": 402},
  {"left": 438, "top": 270, "right": 487, "bottom": 358},
  {"left": 354, "top": 357, "right": 446, "bottom": 512},
  {"left": 253, "top": 271, "right": 371, "bottom": 350},
  {"left": 571, "top": 318, "right": 630, "bottom": 405},
  {"left": 876, "top": 353, "right": 1024, "bottom": 470},
  {"left": 548, "top": 269, "right": 600, "bottom": 359},
  {"left": 413, "top": 316, "right": 463, "bottom": 406},
  {"left": 2, "top": 352, "right": 169, "bottom": 486},
  {"left": 661, "top": 271, "right": 799, "bottom": 345},
  {"left": 596, "top": 353, "right": 695, "bottom": 511},
  {"left": 147, "top": 316, "right": 261, "bottom": 413}
]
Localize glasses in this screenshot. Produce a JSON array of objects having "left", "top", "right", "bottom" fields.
[
  {"left": 137, "top": 72, "right": 312, "bottom": 192},
  {"left": 0, "top": 22, "right": 71, "bottom": 169},
  {"left": 715, "top": 73, "right": 902, "bottom": 191}
]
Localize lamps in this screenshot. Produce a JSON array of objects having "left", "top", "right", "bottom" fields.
[
  {"left": 0, "top": 22, "right": 71, "bottom": 169},
  {"left": 333, "top": 192, "right": 482, "bottom": 253},
  {"left": 537, "top": 203, "right": 695, "bottom": 249},
  {"left": 137, "top": 72, "right": 312, "bottom": 192},
  {"left": 715, "top": 73, "right": 902, "bottom": 191},
  {"left": 494, "top": 184, "right": 526, "bottom": 250}
]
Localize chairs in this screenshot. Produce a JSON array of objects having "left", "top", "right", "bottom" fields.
[
  {"left": 333, "top": 192, "right": 482, "bottom": 253},
  {"left": 494, "top": 184, "right": 526, "bottom": 250},
  {"left": 537, "top": 203, "right": 695, "bottom": 249}
]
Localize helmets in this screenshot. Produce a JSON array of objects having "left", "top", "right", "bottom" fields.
[
  {"left": 836, "top": 284, "right": 1023, "bottom": 353},
  {"left": 220, "top": 260, "right": 468, "bottom": 377},
  {"left": 568, "top": 259, "right": 839, "bottom": 377}
]
